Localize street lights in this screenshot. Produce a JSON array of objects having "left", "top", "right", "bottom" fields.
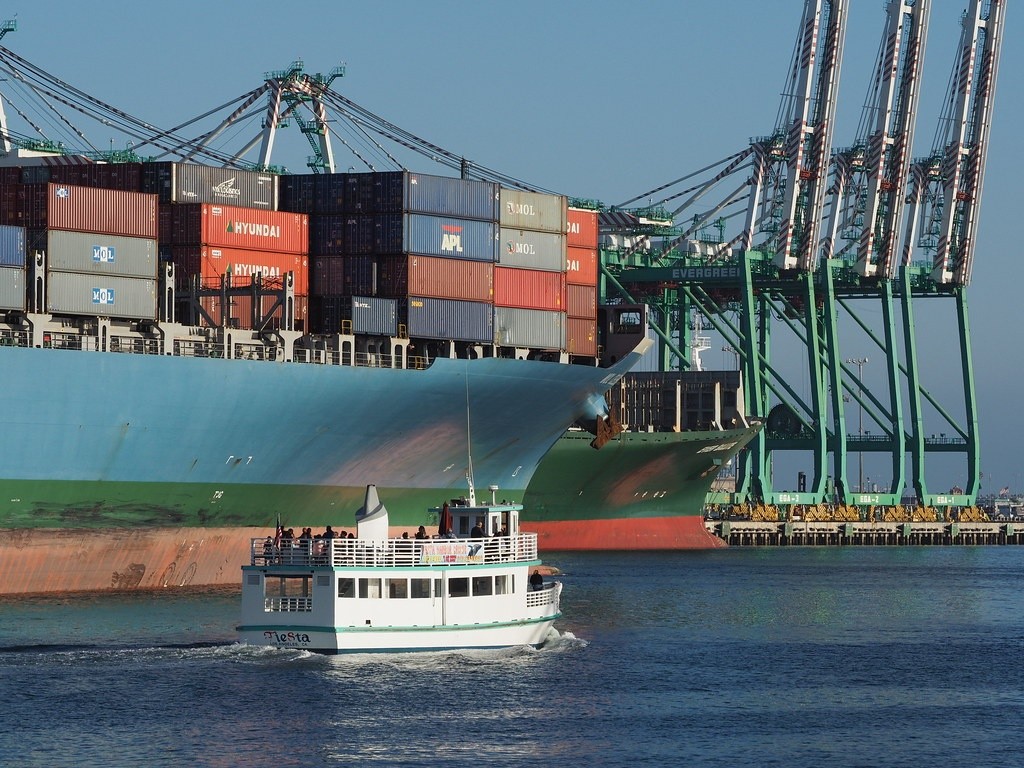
[{"left": 846, "top": 358, "right": 868, "bottom": 491}]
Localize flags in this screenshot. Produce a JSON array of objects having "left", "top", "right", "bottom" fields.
[{"left": 1000, "top": 487, "right": 1009, "bottom": 495}]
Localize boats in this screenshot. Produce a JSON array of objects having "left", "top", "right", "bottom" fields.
[
  {"left": 520, "top": 311, "right": 767, "bottom": 551},
  {"left": 0, "top": 251, "right": 656, "bottom": 648},
  {"left": 236, "top": 368, "right": 563, "bottom": 656}
]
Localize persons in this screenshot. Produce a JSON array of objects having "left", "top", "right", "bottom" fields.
[
  {"left": 399, "top": 532, "right": 411, "bottom": 552},
  {"left": 443, "top": 528, "right": 457, "bottom": 539},
  {"left": 415, "top": 526, "right": 430, "bottom": 539},
  {"left": 471, "top": 522, "right": 489, "bottom": 542},
  {"left": 265, "top": 526, "right": 354, "bottom": 566},
  {"left": 530, "top": 570, "right": 543, "bottom": 590}
]
